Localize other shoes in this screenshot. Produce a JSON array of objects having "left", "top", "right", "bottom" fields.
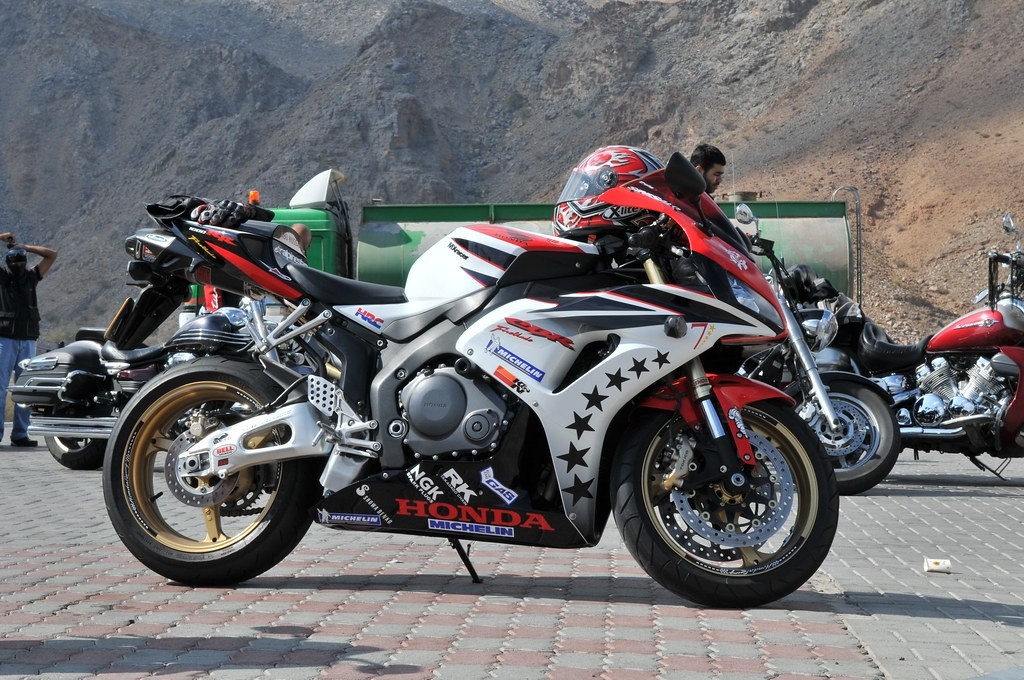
[{"left": 11, "top": 436, "right": 38, "bottom": 447}]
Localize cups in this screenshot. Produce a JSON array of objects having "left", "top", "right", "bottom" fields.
[{"left": 923, "top": 555, "right": 953, "bottom": 574}]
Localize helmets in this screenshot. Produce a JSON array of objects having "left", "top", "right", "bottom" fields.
[{"left": 552, "top": 145, "right": 666, "bottom": 239}]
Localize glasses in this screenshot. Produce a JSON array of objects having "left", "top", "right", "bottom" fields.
[{"left": 7, "top": 249, "right": 27, "bottom": 257}]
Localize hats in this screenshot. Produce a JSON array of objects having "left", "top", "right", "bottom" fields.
[{"left": 6, "top": 247, "right": 27, "bottom": 264}]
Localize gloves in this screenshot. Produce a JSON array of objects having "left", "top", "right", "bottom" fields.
[
  {"left": 191, "top": 199, "right": 275, "bottom": 230},
  {"left": 147, "top": 194, "right": 213, "bottom": 219}
]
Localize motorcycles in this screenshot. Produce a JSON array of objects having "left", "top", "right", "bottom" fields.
[
  {"left": 815, "top": 212, "right": 1024, "bottom": 481},
  {"left": 101, "top": 150, "right": 841, "bottom": 610},
  {"left": 725, "top": 201, "right": 897, "bottom": 496},
  {"left": 6, "top": 189, "right": 325, "bottom": 472}
]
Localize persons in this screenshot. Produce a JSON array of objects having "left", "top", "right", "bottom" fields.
[
  {"left": 291, "top": 223, "right": 311, "bottom": 255},
  {"left": 689, "top": 143, "right": 752, "bottom": 238},
  {"left": 0, "top": 232, "right": 57, "bottom": 446}
]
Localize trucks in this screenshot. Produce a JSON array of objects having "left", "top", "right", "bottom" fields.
[{"left": 176, "top": 168, "right": 860, "bottom": 336}]
